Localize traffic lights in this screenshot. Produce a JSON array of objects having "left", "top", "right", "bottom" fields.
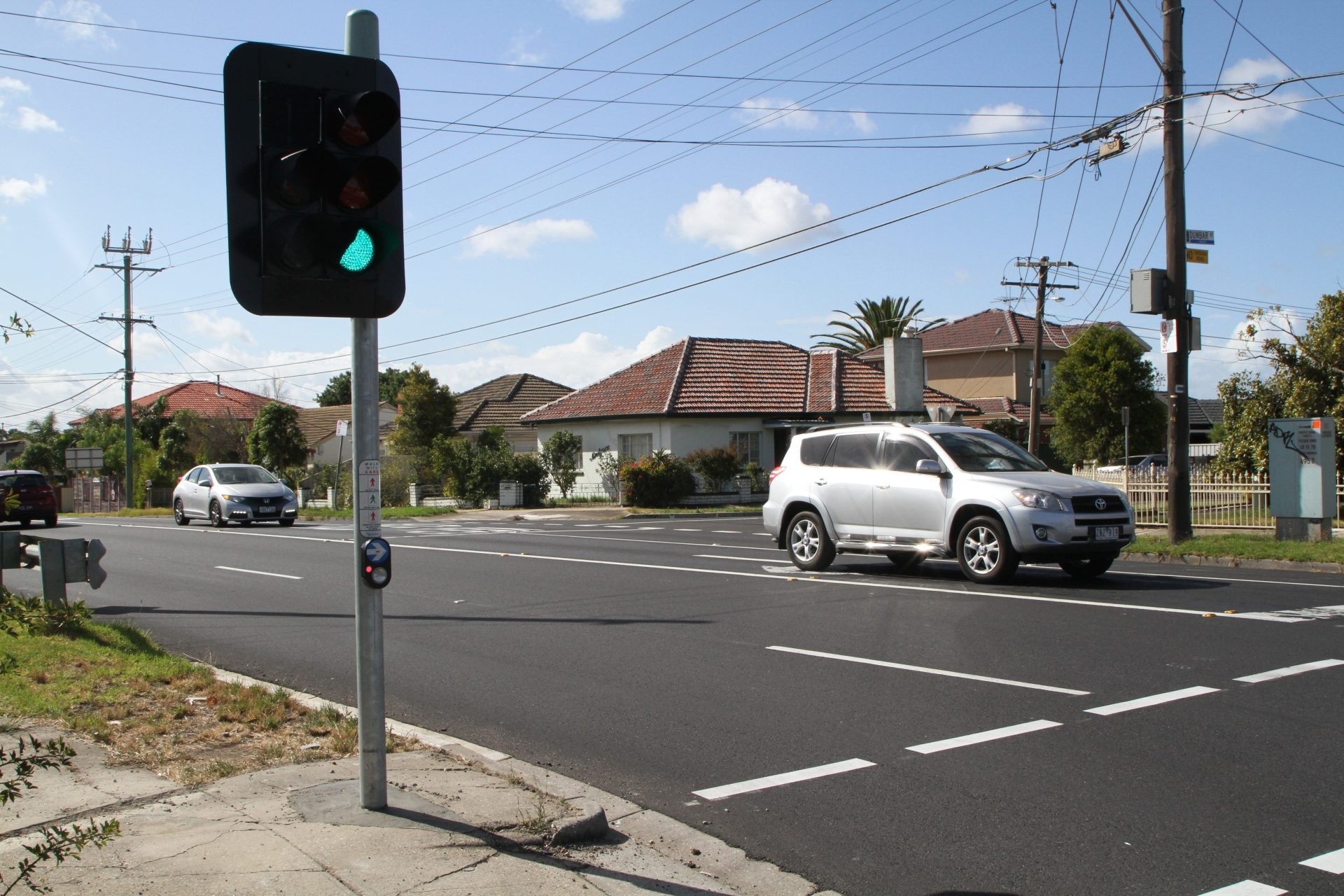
[{"left": 226, "top": 42, "right": 404, "bottom": 318}]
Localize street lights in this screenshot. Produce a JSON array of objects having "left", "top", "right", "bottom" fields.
[{"left": 1028, "top": 297, "right": 1066, "bottom": 469}]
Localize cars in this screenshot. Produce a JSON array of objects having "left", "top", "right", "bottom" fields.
[{"left": 173, "top": 462, "right": 298, "bottom": 528}]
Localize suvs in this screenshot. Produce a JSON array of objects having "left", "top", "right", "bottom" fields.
[
  {"left": 0, "top": 469, "right": 57, "bottom": 527},
  {"left": 763, "top": 421, "right": 1135, "bottom": 584}
]
